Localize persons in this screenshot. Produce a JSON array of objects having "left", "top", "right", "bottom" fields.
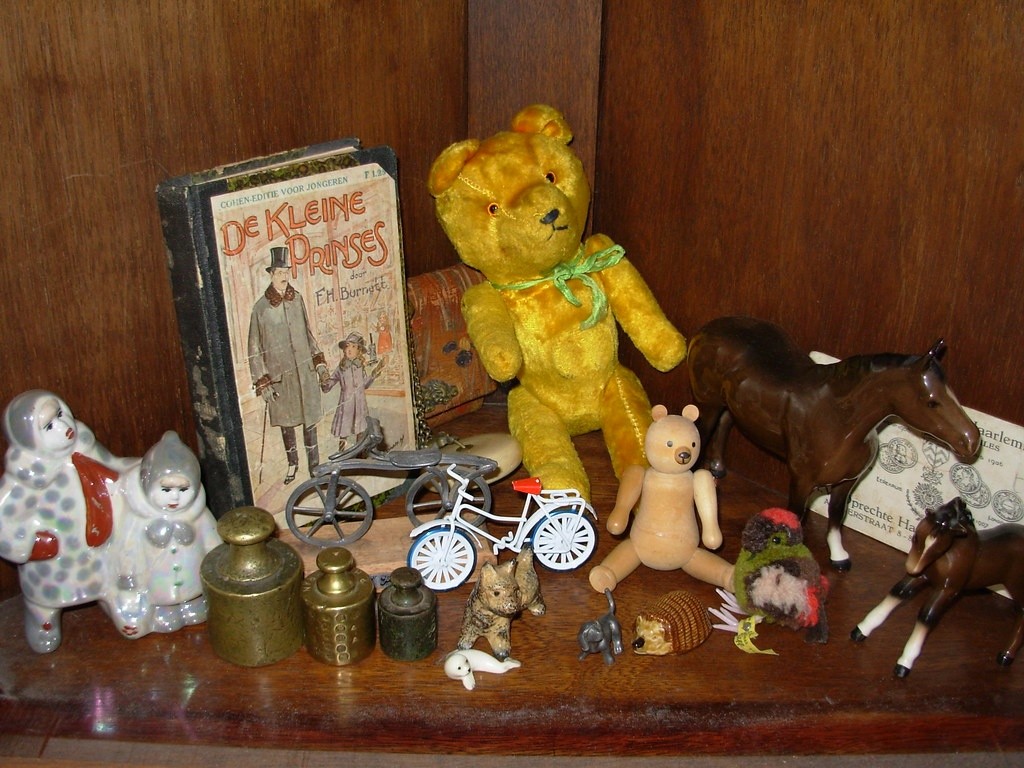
[{"left": 0, "top": 388, "right": 221, "bottom": 656}]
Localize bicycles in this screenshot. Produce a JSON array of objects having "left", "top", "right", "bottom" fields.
[
  {"left": 284, "top": 419, "right": 492, "bottom": 547},
  {"left": 407, "top": 463, "right": 598, "bottom": 593}
]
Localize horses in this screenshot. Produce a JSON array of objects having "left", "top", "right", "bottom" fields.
[
  {"left": 848, "top": 497, "right": 1024, "bottom": 682},
  {"left": 688, "top": 312, "right": 985, "bottom": 571}
]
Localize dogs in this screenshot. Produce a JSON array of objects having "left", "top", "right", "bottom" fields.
[
  {"left": 579, "top": 589, "right": 623, "bottom": 665},
  {"left": 457, "top": 543, "right": 547, "bottom": 659}
]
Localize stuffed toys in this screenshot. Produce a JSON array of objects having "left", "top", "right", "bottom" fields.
[{"left": 429, "top": 104, "right": 687, "bottom": 514}]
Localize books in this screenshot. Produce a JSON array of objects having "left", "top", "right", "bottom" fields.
[{"left": 154, "top": 137, "right": 419, "bottom": 529}]
